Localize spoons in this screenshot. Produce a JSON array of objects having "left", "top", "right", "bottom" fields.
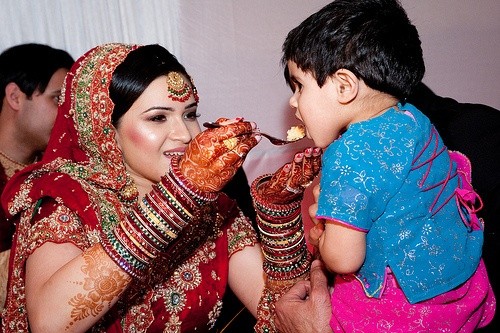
[{"left": 201, "top": 121, "right": 307, "bottom": 146}]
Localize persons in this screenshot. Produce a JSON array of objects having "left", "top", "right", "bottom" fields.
[
  {"left": 273, "top": 0, "right": 500, "bottom": 333},
  {"left": 0, "top": 41, "right": 74, "bottom": 308},
  {"left": 2, "top": 42, "right": 324, "bottom": 332}
]
